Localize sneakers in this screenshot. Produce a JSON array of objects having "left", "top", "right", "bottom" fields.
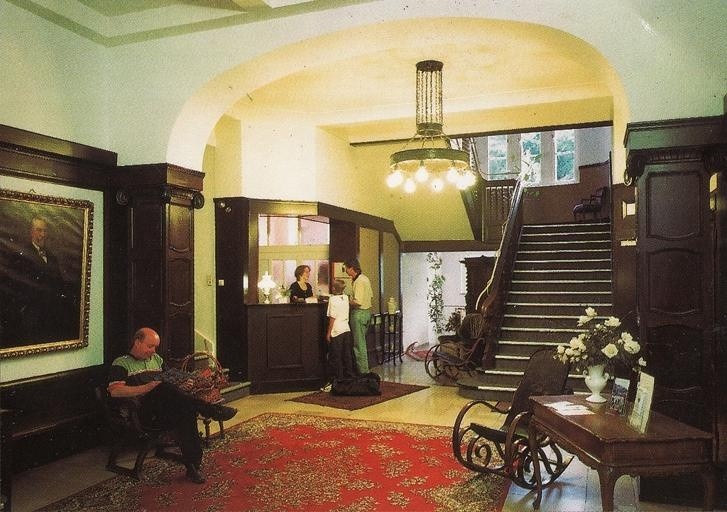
[
  {"left": 211, "top": 405, "right": 237, "bottom": 421},
  {"left": 320, "top": 383, "right": 332, "bottom": 393},
  {"left": 186, "top": 463, "right": 206, "bottom": 483}
]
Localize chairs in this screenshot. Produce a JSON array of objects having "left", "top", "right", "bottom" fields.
[
  {"left": 424, "top": 313, "right": 485, "bottom": 382},
  {"left": 451, "top": 349, "right": 576, "bottom": 492},
  {"left": 573, "top": 187, "right": 608, "bottom": 222},
  {"left": 93, "top": 384, "right": 178, "bottom": 481}
]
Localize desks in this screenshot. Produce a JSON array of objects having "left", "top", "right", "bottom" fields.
[{"left": 527, "top": 393, "right": 718, "bottom": 511}]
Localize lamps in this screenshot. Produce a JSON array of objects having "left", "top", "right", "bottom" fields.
[
  {"left": 257, "top": 271, "right": 278, "bottom": 304},
  {"left": 389, "top": 59, "right": 477, "bottom": 192}
]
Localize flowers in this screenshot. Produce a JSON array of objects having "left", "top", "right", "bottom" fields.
[{"left": 554, "top": 307, "right": 646, "bottom": 370}]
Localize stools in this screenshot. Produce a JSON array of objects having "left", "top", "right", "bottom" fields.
[
  {"left": 194, "top": 398, "right": 227, "bottom": 451},
  {"left": 379, "top": 311, "right": 403, "bottom": 366}
]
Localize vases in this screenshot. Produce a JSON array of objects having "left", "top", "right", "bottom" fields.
[{"left": 583, "top": 364, "right": 610, "bottom": 403}]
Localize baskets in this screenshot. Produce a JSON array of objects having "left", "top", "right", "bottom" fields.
[{"left": 182, "top": 352, "right": 222, "bottom": 403}]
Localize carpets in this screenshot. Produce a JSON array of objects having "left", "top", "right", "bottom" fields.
[
  {"left": 35, "top": 412, "right": 525, "bottom": 512},
  {"left": 406, "top": 341, "right": 434, "bottom": 360},
  {"left": 285, "top": 379, "right": 430, "bottom": 412}
]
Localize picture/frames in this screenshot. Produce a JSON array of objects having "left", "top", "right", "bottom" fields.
[{"left": 1, "top": 187, "right": 95, "bottom": 358}]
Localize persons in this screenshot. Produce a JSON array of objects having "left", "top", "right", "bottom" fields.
[
  {"left": 343, "top": 256, "right": 374, "bottom": 377},
  {"left": 14, "top": 212, "right": 68, "bottom": 289},
  {"left": 286, "top": 264, "right": 314, "bottom": 303},
  {"left": 319, "top": 279, "right": 355, "bottom": 394},
  {"left": 103, "top": 326, "right": 239, "bottom": 483}
]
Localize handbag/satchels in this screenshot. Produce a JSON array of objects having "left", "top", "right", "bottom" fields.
[{"left": 332, "top": 372, "right": 382, "bottom": 396}]
